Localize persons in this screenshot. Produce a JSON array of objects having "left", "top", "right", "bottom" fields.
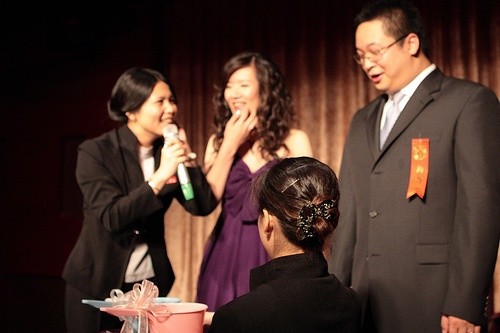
[
  {"left": 327, "top": 1, "right": 500, "bottom": 333},
  {"left": 208, "top": 156, "right": 363, "bottom": 333},
  {"left": 63, "top": 65, "right": 218, "bottom": 333},
  {"left": 194, "top": 52, "right": 314, "bottom": 311}
]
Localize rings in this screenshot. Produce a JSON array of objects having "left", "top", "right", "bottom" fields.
[{"left": 163, "top": 144, "right": 167, "bottom": 149}]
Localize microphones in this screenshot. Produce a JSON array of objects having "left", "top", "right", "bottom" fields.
[{"left": 162, "top": 123, "right": 194, "bottom": 200}]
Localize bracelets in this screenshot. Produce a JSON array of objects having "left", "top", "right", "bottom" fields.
[
  {"left": 187, "top": 152, "right": 198, "bottom": 160},
  {"left": 148, "top": 179, "right": 161, "bottom": 194}
]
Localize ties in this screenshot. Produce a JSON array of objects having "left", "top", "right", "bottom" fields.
[{"left": 380, "top": 92, "right": 405, "bottom": 150}]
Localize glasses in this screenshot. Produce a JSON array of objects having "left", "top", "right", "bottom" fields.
[{"left": 354, "top": 33, "right": 408, "bottom": 64}]
